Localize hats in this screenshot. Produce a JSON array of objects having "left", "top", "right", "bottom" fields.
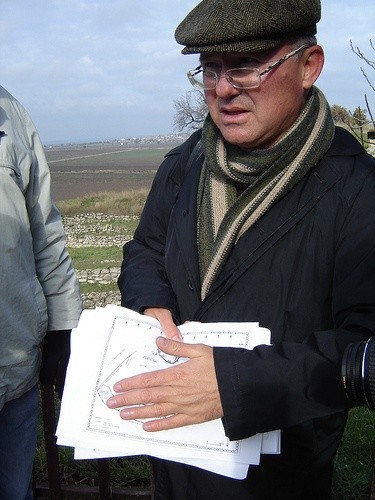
[{"left": 174, "top": 0, "right": 321, "bottom": 55}]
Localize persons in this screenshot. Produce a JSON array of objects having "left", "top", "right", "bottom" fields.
[
  {"left": 108, "top": 0, "right": 374, "bottom": 500},
  {"left": 0, "top": 84, "right": 84, "bottom": 500}
]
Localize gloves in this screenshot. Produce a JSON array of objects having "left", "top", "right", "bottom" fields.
[{"left": 40, "top": 330, "right": 70, "bottom": 400}]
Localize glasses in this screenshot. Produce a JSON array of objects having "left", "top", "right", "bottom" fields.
[{"left": 186, "top": 44, "right": 311, "bottom": 90}]
